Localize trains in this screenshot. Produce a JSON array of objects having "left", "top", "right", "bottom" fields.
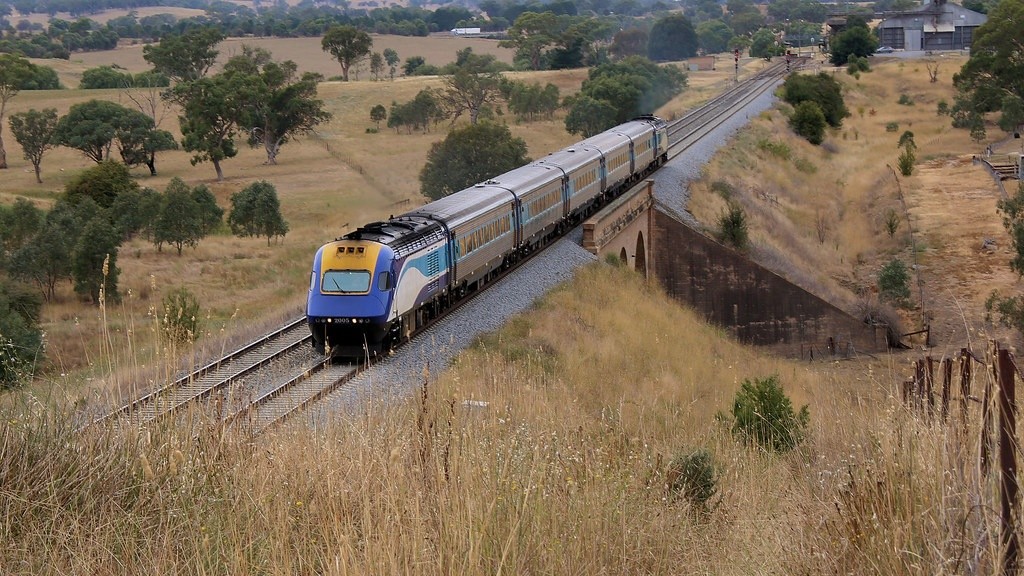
[{"left": 303, "top": 112, "right": 668, "bottom": 359}]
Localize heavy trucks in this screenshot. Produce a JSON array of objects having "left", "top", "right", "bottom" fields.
[{"left": 451, "top": 28, "right": 480, "bottom": 35}]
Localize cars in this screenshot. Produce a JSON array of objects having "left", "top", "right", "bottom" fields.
[{"left": 876, "top": 46, "right": 895, "bottom": 53}]
